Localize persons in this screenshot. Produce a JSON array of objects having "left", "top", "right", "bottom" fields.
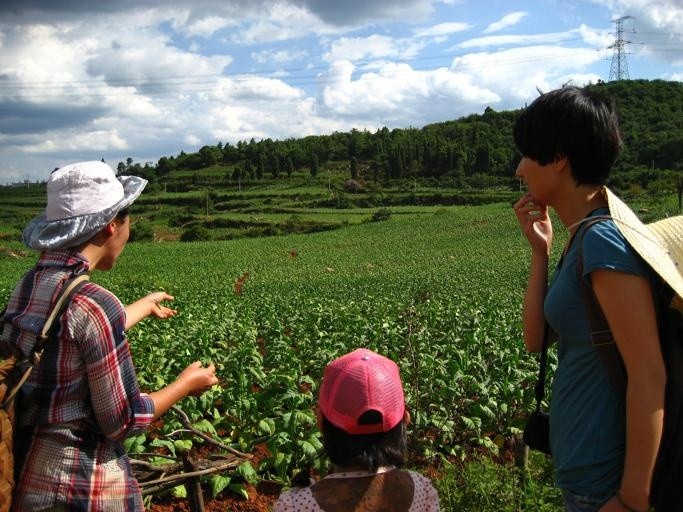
[
  {"left": 0, "top": 159, "right": 219, "bottom": 512},
  {"left": 513, "top": 84, "right": 681, "bottom": 509},
  {"left": 268, "top": 346, "right": 441, "bottom": 510}
]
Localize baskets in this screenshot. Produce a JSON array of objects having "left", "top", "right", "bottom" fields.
[{"left": 0, "top": 338, "right": 30, "bottom": 510}]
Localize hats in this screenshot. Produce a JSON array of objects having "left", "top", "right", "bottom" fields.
[
  {"left": 316, "top": 346, "right": 407, "bottom": 438},
  {"left": 602, "top": 178, "right": 683, "bottom": 301},
  {"left": 19, "top": 158, "right": 148, "bottom": 254}
]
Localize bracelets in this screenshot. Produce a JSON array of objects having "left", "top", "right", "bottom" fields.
[{"left": 614, "top": 488, "right": 654, "bottom": 511}]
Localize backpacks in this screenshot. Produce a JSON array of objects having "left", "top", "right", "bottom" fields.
[{"left": 577, "top": 218, "right": 681, "bottom": 510}]
